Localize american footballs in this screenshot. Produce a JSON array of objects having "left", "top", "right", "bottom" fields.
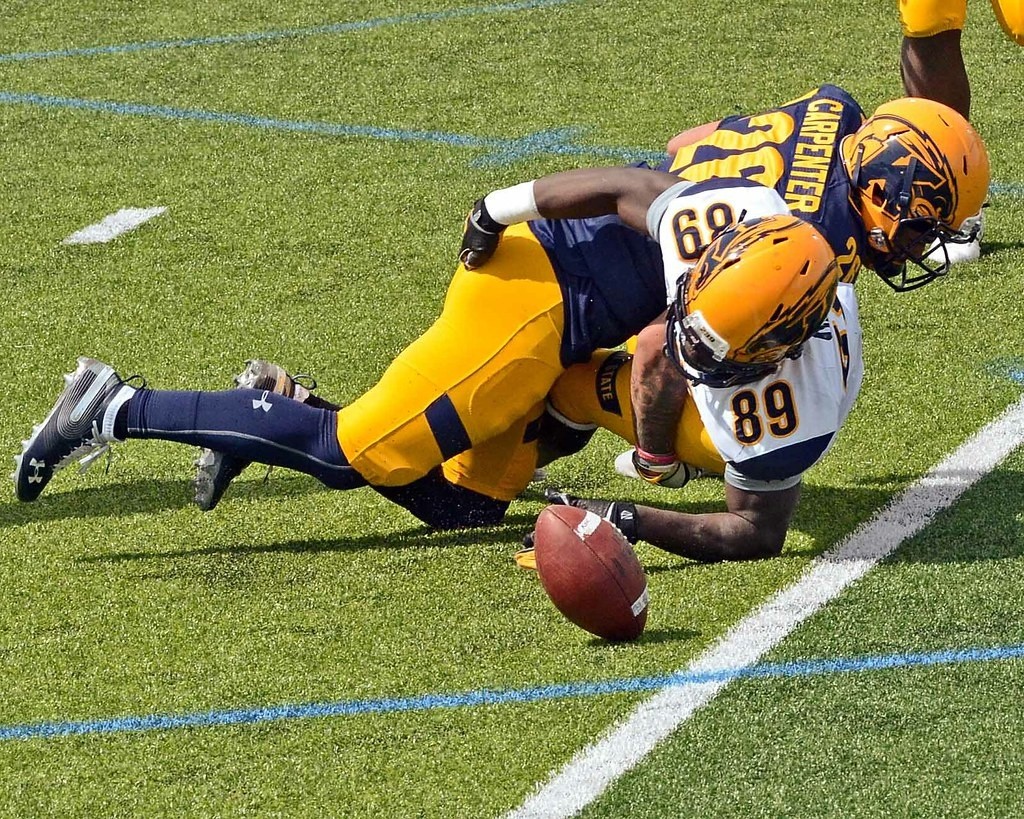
[{"left": 532, "top": 502, "right": 650, "bottom": 645}]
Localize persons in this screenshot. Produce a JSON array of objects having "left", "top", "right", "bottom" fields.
[
  {"left": 895, "top": 1, "right": 1024, "bottom": 264},
  {"left": 458, "top": 164, "right": 862, "bottom": 559},
  {"left": 14, "top": 84, "right": 993, "bottom": 531}
]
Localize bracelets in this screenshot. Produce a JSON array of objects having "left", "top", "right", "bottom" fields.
[
  {"left": 636, "top": 447, "right": 678, "bottom": 465},
  {"left": 472, "top": 197, "right": 509, "bottom": 234}
]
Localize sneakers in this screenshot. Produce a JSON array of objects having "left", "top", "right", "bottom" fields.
[
  {"left": 192, "top": 358, "right": 296, "bottom": 512},
  {"left": 613, "top": 449, "right": 639, "bottom": 479},
  {"left": 9, "top": 356, "right": 119, "bottom": 504}
]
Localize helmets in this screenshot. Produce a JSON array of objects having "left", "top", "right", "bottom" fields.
[
  {"left": 660, "top": 213, "right": 851, "bottom": 389},
  {"left": 838, "top": 96, "right": 990, "bottom": 295}
]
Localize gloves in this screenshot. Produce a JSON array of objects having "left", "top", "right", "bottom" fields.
[
  {"left": 522, "top": 486, "right": 641, "bottom": 546},
  {"left": 457, "top": 193, "right": 510, "bottom": 272},
  {"left": 631, "top": 452, "right": 704, "bottom": 489}
]
